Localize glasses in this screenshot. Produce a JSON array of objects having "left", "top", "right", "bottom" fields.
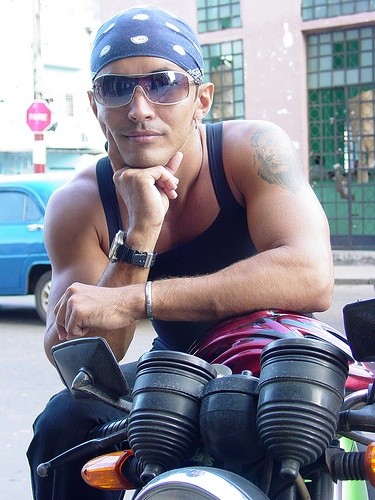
[{"left": 92, "top": 71, "right": 201, "bottom": 108}]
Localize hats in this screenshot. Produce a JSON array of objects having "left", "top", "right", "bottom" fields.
[{"left": 90, "top": 7, "right": 203, "bottom": 86}]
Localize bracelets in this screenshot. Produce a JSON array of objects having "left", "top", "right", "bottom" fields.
[{"left": 144, "top": 281, "right": 155, "bottom": 322}]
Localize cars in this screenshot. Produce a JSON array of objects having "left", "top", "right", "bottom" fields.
[{"left": 0, "top": 172, "right": 74, "bottom": 322}]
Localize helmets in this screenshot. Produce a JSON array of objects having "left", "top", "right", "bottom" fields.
[{"left": 187, "top": 309, "right": 375, "bottom": 392}]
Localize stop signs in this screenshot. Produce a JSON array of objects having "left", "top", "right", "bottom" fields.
[{"left": 26, "top": 100, "right": 53, "bottom": 132}]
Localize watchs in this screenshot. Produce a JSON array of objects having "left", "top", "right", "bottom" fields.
[{"left": 108, "top": 229, "right": 157, "bottom": 269}]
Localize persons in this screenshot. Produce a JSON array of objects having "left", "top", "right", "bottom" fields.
[{"left": 26, "top": 10, "right": 333, "bottom": 500}]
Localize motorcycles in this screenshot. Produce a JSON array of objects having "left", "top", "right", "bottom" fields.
[{"left": 36, "top": 299, "right": 375, "bottom": 500}]
